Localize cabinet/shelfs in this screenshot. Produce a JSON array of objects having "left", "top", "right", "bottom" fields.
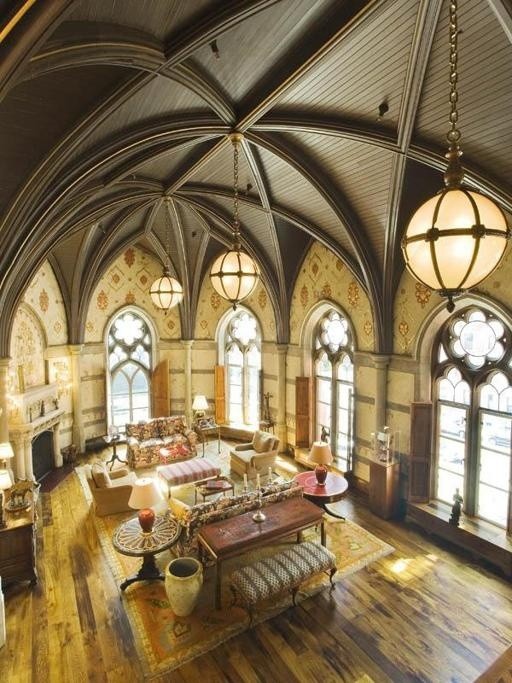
[
  {"left": 2, "top": 488, "right": 37, "bottom": 594},
  {"left": 368, "top": 454, "right": 402, "bottom": 522}
]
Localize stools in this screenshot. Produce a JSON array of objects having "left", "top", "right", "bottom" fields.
[{"left": 194, "top": 477, "right": 236, "bottom": 506}]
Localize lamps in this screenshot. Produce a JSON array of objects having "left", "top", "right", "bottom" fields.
[
  {"left": 309, "top": 442, "right": 334, "bottom": 485},
  {"left": 1, "top": 470, "right": 14, "bottom": 509},
  {"left": 150, "top": 194, "right": 184, "bottom": 316},
  {"left": 128, "top": 477, "right": 163, "bottom": 534},
  {"left": 192, "top": 396, "right": 209, "bottom": 420},
  {"left": 208, "top": 126, "right": 261, "bottom": 311},
  {"left": 0, "top": 443, "right": 15, "bottom": 470},
  {"left": 401, "top": 4, "right": 508, "bottom": 314}
]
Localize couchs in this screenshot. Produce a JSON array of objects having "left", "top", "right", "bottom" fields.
[
  {"left": 126, "top": 415, "right": 200, "bottom": 470},
  {"left": 230, "top": 430, "right": 281, "bottom": 481},
  {"left": 84, "top": 463, "right": 141, "bottom": 518}
]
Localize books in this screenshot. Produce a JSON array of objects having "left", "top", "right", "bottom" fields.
[{"left": 206, "top": 481, "right": 222, "bottom": 490}]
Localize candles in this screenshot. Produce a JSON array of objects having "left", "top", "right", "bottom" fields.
[
  {"left": 257, "top": 475, "right": 261, "bottom": 489},
  {"left": 244, "top": 473, "right": 248, "bottom": 488},
  {"left": 269, "top": 467, "right": 272, "bottom": 480}
]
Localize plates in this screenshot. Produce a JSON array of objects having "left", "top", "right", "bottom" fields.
[{"left": 253, "top": 514, "right": 266, "bottom": 521}]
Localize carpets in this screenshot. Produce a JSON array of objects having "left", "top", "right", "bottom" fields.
[{"left": 75, "top": 440, "right": 394, "bottom": 681}]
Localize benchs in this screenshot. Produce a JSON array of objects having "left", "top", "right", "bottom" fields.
[{"left": 230, "top": 541, "right": 338, "bottom": 635}]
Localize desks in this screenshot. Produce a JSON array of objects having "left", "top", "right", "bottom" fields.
[{"left": 194, "top": 497, "right": 328, "bottom": 611}]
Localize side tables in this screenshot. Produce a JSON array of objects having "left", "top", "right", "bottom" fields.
[
  {"left": 294, "top": 471, "right": 349, "bottom": 530},
  {"left": 194, "top": 421, "right": 222, "bottom": 458},
  {"left": 102, "top": 434, "right": 129, "bottom": 472},
  {"left": 114, "top": 516, "right": 184, "bottom": 593}
]
